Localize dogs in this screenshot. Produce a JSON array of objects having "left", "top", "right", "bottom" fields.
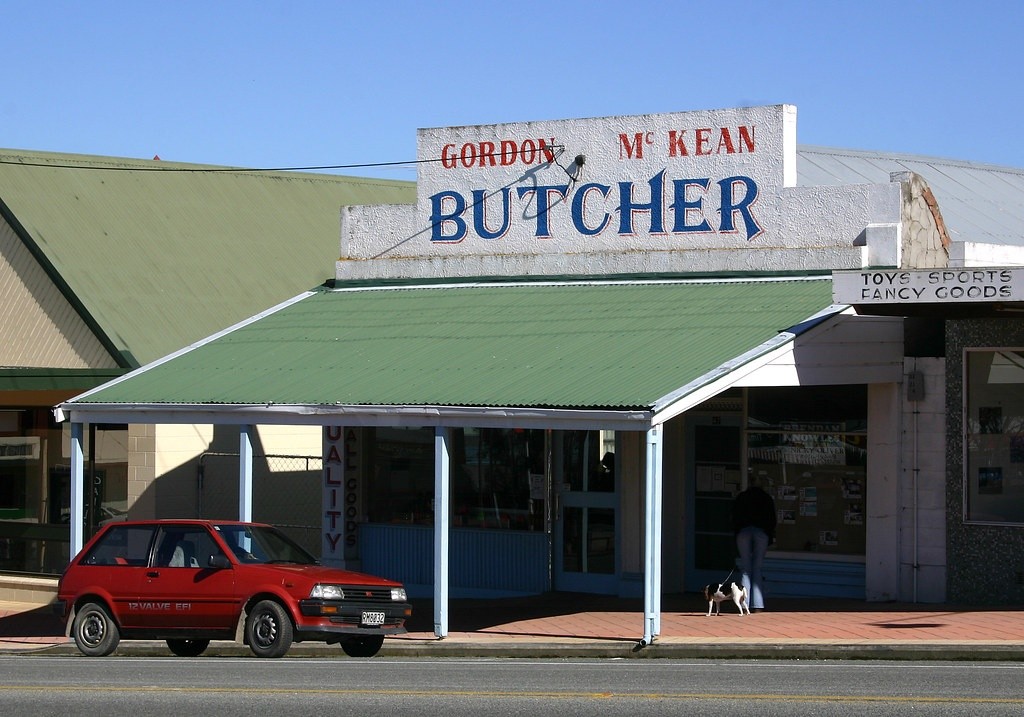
[{"left": 700, "top": 581, "right": 751, "bottom": 616}]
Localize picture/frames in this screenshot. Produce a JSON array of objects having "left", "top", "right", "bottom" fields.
[
  {"left": 978, "top": 407, "right": 1003, "bottom": 434},
  {"left": 978, "top": 467, "right": 1003, "bottom": 495}
]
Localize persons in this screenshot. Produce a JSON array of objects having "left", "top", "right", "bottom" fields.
[
  {"left": 596, "top": 452, "right": 615, "bottom": 492},
  {"left": 732, "top": 483, "right": 776, "bottom": 615}
]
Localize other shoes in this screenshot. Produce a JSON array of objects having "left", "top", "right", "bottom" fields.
[{"left": 751, "top": 608, "right": 768, "bottom": 612}]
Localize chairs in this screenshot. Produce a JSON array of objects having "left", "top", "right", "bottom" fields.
[
  {"left": 145, "top": 540, "right": 186, "bottom": 567},
  {"left": 182, "top": 541, "right": 196, "bottom": 566},
  {"left": 107, "top": 556, "right": 135, "bottom": 566},
  {"left": 199, "top": 536, "right": 216, "bottom": 568}
]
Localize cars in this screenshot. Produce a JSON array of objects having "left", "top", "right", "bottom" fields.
[{"left": 56, "top": 516, "right": 414, "bottom": 660}]
